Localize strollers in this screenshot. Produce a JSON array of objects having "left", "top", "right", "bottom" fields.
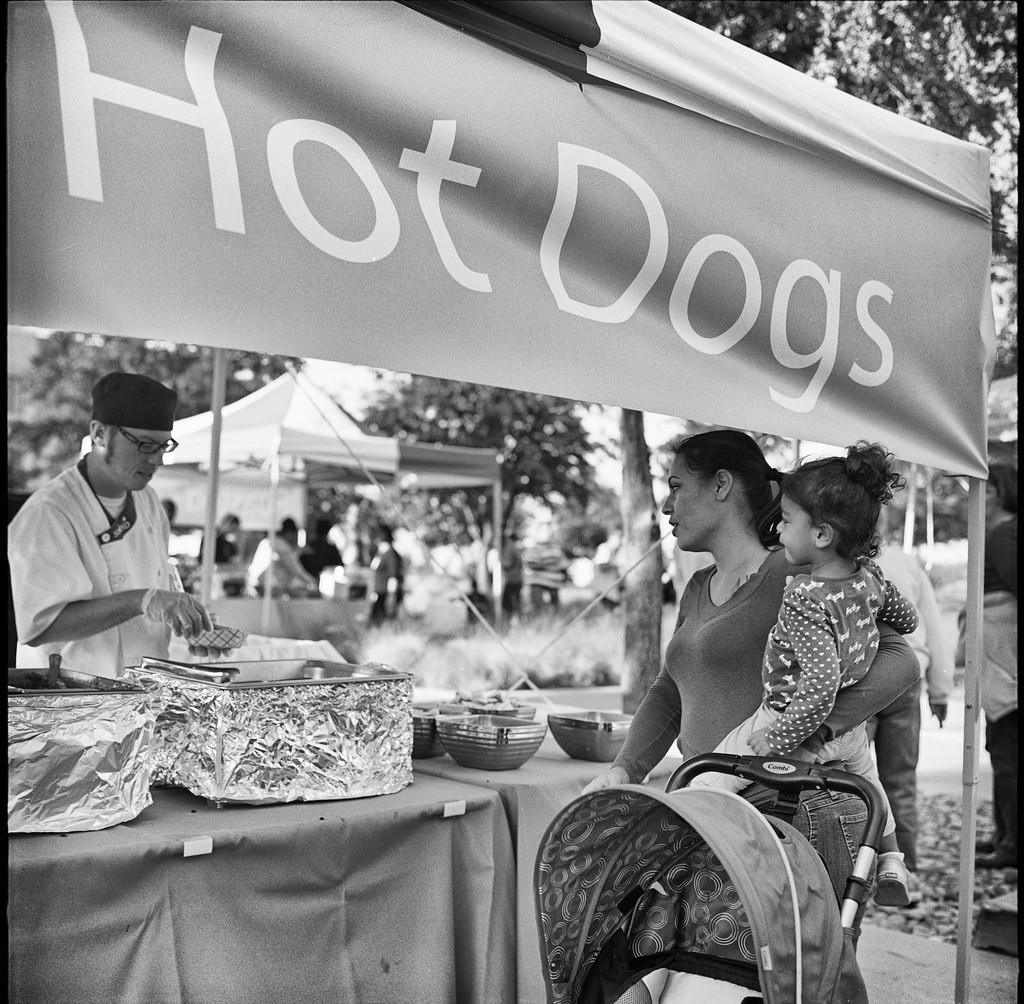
[{"left": 533, "top": 750, "right": 889, "bottom": 1004}]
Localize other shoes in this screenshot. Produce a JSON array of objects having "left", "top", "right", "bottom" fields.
[
  {"left": 907, "top": 872, "right": 922, "bottom": 903},
  {"left": 975, "top": 851, "right": 1017, "bottom": 869},
  {"left": 975, "top": 839, "right": 995, "bottom": 854}
]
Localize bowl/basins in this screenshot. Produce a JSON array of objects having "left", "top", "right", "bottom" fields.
[
  {"left": 465, "top": 704, "right": 536, "bottom": 720},
  {"left": 437, "top": 714, "right": 547, "bottom": 771},
  {"left": 548, "top": 711, "right": 639, "bottom": 762},
  {"left": 411, "top": 706, "right": 462, "bottom": 760}
]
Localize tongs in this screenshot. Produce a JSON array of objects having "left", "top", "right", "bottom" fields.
[{"left": 142, "top": 656, "right": 240, "bottom": 684}]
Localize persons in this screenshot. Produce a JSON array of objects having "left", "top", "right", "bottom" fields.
[
  {"left": 7, "top": 373, "right": 239, "bottom": 686},
  {"left": 974, "top": 463, "right": 1020, "bottom": 874},
  {"left": 577, "top": 429, "right": 925, "bottom": 1003},
  {"left": 672, "top": 438, "right": 924, "bottom": 907},
  {"left": 158, "top": 493, "right": 569, "bottom": 632},
  {"left": 863, "top": 543, "right": 954, "bottom": 905}
]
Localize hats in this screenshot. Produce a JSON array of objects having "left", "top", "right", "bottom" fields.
[{"left": 92, "top": 371, "right": 178, "bottom": 430}]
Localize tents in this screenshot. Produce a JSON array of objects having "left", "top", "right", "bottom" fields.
[
  {"left": 79, "top": 369, "right": 507, "bottom": 631},
  {"left": 900, "top": 370, "right": 1019, "bottom": 571},
  {"left": 7, "top": 1, "right": 994, "bottom": 1003}
]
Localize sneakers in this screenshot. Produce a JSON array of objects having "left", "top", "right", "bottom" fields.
[{"left": 873, "top": 856, "right": 911, "bottom": 906}]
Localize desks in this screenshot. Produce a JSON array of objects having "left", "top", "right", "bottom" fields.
[{"left": 8, "top": 597, "right": 685, "bottom": 1004}]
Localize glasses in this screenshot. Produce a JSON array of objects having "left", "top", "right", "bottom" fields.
[{"left": 117, "top": 426, "right": 179, "bottom": 454}]
